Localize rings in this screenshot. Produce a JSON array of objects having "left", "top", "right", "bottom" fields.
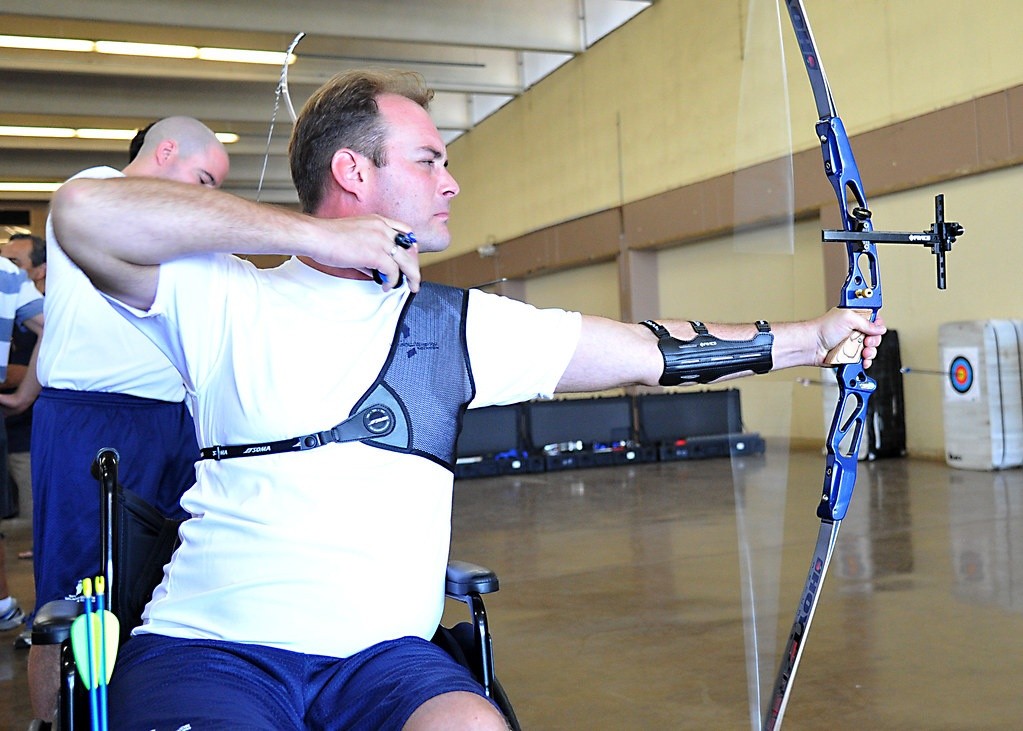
[{"left": 388, "top": 243, "right": 398, "bottom": 257}]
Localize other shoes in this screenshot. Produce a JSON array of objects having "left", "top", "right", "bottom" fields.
[{"left": 0, "top": 598, "right": 26, "bottom": 631}]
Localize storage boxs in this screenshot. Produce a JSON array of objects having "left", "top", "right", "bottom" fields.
[{"left": 454, "top": 390, "right": 765, "bottom": 479}]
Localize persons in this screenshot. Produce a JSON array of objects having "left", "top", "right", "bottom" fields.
[
  {"left": 46, "top": 63, "right": 890, "bottom": 731},
  {"left": 30, "top": 111, "right": 236, "bottom": 646},
  {"left": 1, "top": 231, "right": 49, "bottom": 650}
]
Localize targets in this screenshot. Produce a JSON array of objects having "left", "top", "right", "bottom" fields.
[{"left": 943, "top": 346, "right": 980, "bottom": 402}]
